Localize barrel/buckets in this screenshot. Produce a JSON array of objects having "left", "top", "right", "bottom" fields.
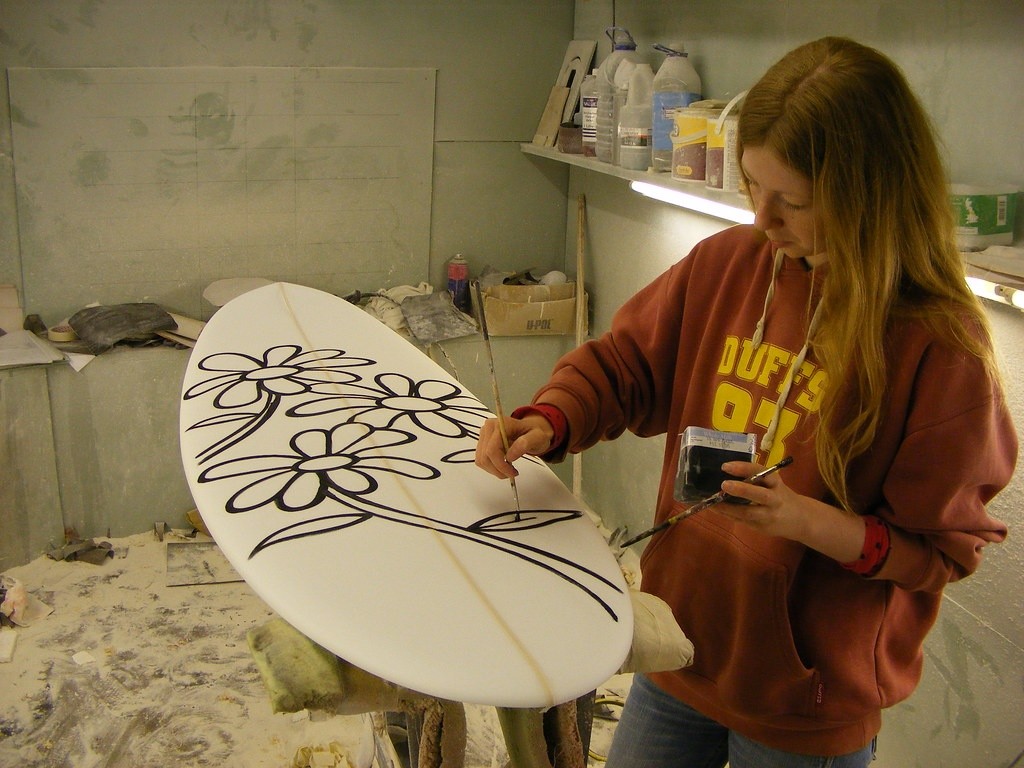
[{"left": 669, "top": 89, "right": 749, "bottom": 194}]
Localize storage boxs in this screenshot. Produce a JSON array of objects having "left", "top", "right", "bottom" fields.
[
  {"left": 484, "top": 271, "right": 576, "bottom": 303},
  {"left": 469, "top": 278, "right": 589, "bottom": 336}
]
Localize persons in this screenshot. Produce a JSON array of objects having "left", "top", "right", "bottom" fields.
[{"left": 475, "top": 36, "right": 1019, "bottom": 768}]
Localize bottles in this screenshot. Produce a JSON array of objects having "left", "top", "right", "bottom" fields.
[
  {"left": 447, "top": 252, "right": 472, "bottom": 313},
  {"left": 579, "top": 27, "right": 702, "bottom": 173}
]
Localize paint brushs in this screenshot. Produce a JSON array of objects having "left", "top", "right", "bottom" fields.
[
  {"left": 619, "top": 454, "right": 793, "bottom": 559},
  {"left": 467, "top": 280, "right": 531, "bottom": 522}
]
